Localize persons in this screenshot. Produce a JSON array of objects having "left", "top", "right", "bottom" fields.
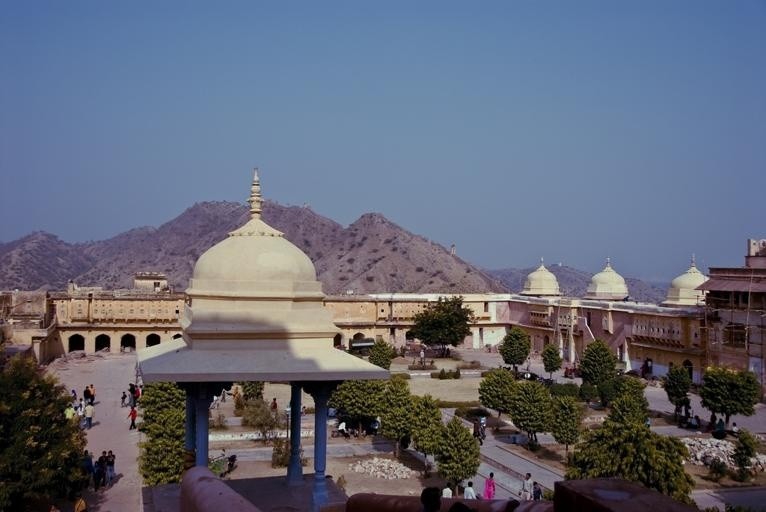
[
  {"left": 328, "top": 404, "right": 338, "bottom": 418},
  {"left": 451, "top": 501, "right": 469, "bottom": 511},
  {"left": 526, "top": 356, "right": 532, "bottom": 371},
  {"left": 221, "top": 391, "right": 226, "bottom": 402},
  {"left": 711, "top": 413, "right": 717, "bottom": 426},
  {"left": 534, "top": 482, "right": 544, "bottom": 500},
  {"left": 372, "top": 415, "right": 381, "bottom": 436},
  {"left": 270, "top": 398, "right": 280, "bottom": 418},
  {"left": 732, "top": 422, "right": 738, "bottom": 432},
  {"left": 418, "top": 487, "right": 441, "bottom": 511},
  {"left": 441, "top": 481, "right": 453, "bottom": 499},
  {"left": 484, "top": 472, "right": 495, "bottom": 500},
  {"left": 212, "top": 397, "right": 218, "bottom": 409},
  {"left": 519, "top": 473, "right": 534, "bottom": 500},
  {"left": 563, "top": 367, "right": 576, "bottom": 382},
  {"left": 220, "top": 448, "right": 226, "bottom": 457},
  {"left": 64, "top": 383, "right": 98, "bottom": 430},
  {"left": 234, "top": 393, "right": 240, "bottom": 403},
  {"left": 644, "top": 416, "right": 652, "bottom": 430},
  {"left": 473, "top": 417, "right": 484, "bottom": 446},
  {"left": 300, "top": 404, "right": 307, "bottom": 416},
  {"left": 718, "top": 418, "right": 724, "bottom": 428},
  {"left": 464, "top": 481, "right": 477, "bottom": 500},
  {"left": 85, "top": 448, "right": 115, "bottom": 490},
  {"left": 338, "top": 421, "right": 348, "bottom": 440},
  {"left": 122, "top": 383, "right": 143, "bottom": 428},
  {"left": 420, "top": 350, "right": 428, "bottom": 366},
  {"left": 480, "top": 412, "right": 487, "bottom": 430},
  {"left": 233, "top": 386, "right": 238, "bottom": 396}
]
